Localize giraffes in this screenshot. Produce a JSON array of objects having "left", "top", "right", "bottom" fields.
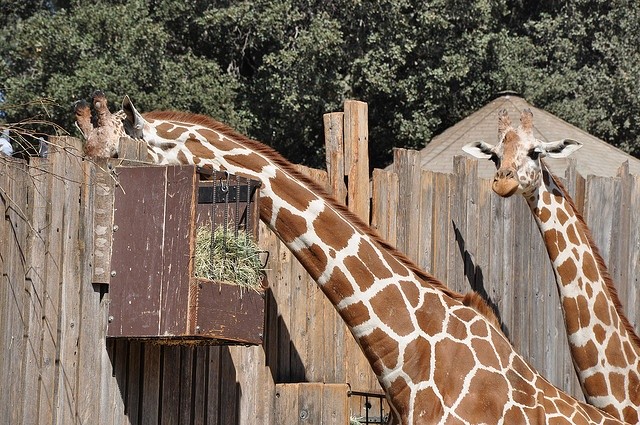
[
  {"left": 460, "top": 105, "right": 640, "bottom": 425},
  {"left": 73, "top": 88, "right": 633, "bottom": 425}
]
